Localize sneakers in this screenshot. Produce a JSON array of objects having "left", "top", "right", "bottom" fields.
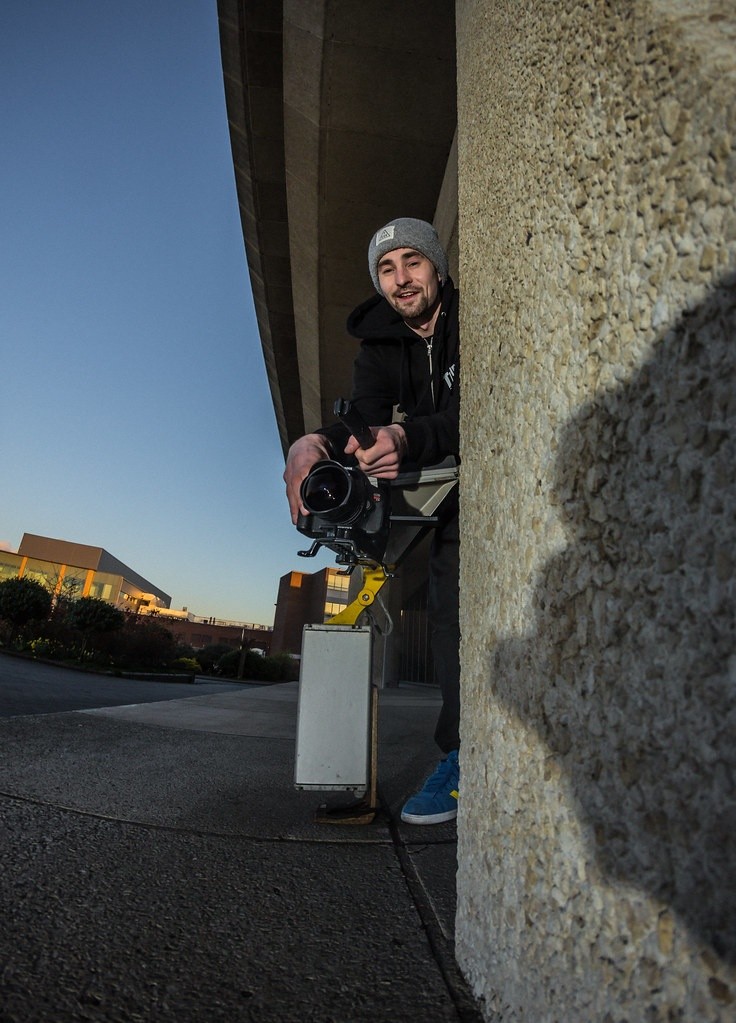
[{"left": 400, "top": 749, "right": 460, "bottom": 823}]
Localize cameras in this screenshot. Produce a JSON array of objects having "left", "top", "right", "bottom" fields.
[{"left": 296, "top": 459, "right": 393, "bottom": 572}]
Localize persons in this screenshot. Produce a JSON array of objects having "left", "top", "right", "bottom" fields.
[{"left": 283, "top": 217, "right": 459, "bottom": 827}]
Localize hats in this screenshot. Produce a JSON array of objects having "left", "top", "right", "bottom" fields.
[{"left": 368, "top": 216, "right": 448, "bottom": 297}]
